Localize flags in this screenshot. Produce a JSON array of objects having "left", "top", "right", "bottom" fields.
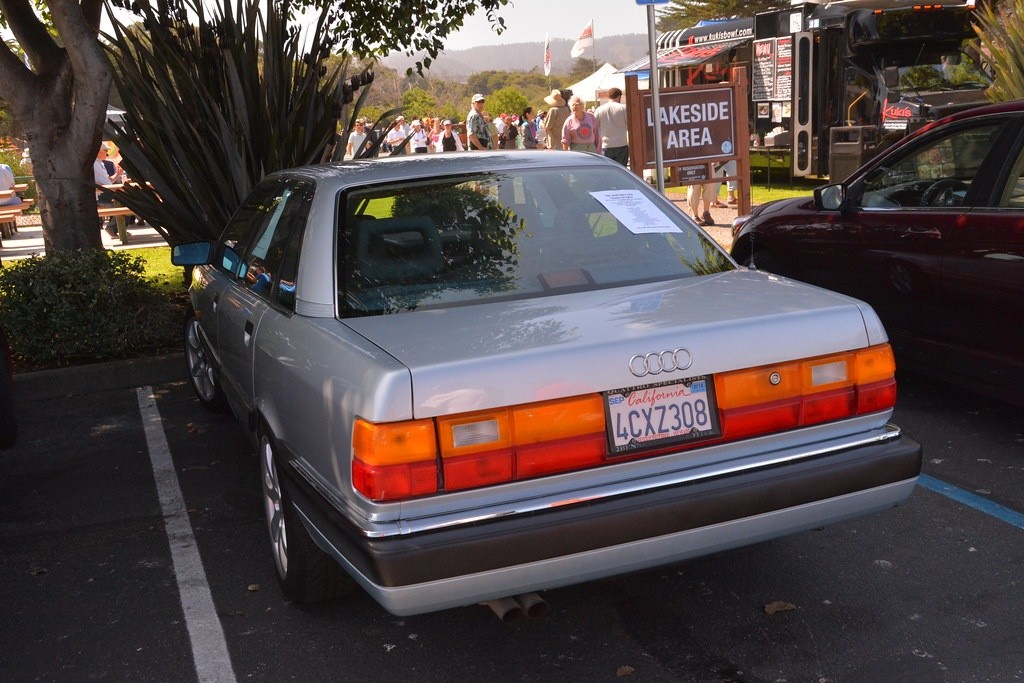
[
  {"left": 571, "top": 18, "right": 594, "bottom": 58},
  {"left": 544, "top": 32, "right": 551, "bottom": 78}
]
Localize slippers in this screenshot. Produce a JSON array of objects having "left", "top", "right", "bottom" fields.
[
  {"left": 726, "top": 198, "right": 738, "bottom": 204},
  {"left": 710, "top": 201, "right": 728, "bottom": 208}
]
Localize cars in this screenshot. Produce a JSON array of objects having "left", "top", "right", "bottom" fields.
[
  {"left": 171, "top": 149, "right": 922, "bottom": 625},
  {"left": 729, "top": 102, "right": 1023, "bottom": 389}
]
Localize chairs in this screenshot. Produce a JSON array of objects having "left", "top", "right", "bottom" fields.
[
  {"left": 346, "top": 213, "right": 448, "bottom": 284},
  {"left": 471, "top": 203, "right": 537, "bottom": 261}
]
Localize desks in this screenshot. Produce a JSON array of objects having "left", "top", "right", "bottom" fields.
[{"left": 97, "top": 181, "right": 152, "bottom": 244}]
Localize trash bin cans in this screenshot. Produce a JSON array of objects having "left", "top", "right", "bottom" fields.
[{"left": 829, "top": 125, "right": 878, "bottom": 191}]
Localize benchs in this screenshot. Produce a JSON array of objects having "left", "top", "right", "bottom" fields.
[{"left": 0, "top": 199, "right": 133, "bottom": 246}]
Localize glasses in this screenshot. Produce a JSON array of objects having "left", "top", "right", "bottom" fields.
[{"left": 476, "top": 100, "right": 485, "bottom": 104}]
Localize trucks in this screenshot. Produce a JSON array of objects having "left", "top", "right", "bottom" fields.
[{"left": 612, "top": 0, "right": 992, "bottom": 176}]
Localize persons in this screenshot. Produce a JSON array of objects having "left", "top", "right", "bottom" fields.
[
  {"left": 323, "top": 82, "right": 630, "bottom": 171},
  {"left": 687, "top": 81, "right": 739, "bottom": 226},
  {"left": 93, "top": 141, "right": 147, "bottom": 240},
  {"left": 0, "top": 164, "right": 22, "bottom": 207}
]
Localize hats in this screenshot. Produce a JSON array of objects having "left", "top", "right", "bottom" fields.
[
  {"left": 544, "top": 89, "right": 566, "bottom": 107},
  {"left": 410, "top": 120, "right": 421, "bottom": 128},
  {"left": 21, "top": 147, "right": 31, "bottom": 158},
  {"left": 395, "top": 116, "right": 405, "bottom": 121},
  {"left": 444, "top": 120, "right": 452, "bottom": 126},
  {"left": 99, "top": 143, "right": 111, "bottom": 151},
  {"left": 472, "top": 93, "right": 484, "bottom": 103}
]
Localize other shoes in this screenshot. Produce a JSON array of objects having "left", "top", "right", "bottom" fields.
[
  {"left": 701, "top": 214, "right": 715, "bottom": 225},
  {"left": 691, "top": 217, "right": 704, "bottom": 226},
  {"left": 127, "top": 218, "right": 135, "bottom": 224},
  {"left": 137, "top": 221, "right": 146, "bottom": 225},
  {"left": 105, "top": 226, "right": 120, "bottom": 239}
]
